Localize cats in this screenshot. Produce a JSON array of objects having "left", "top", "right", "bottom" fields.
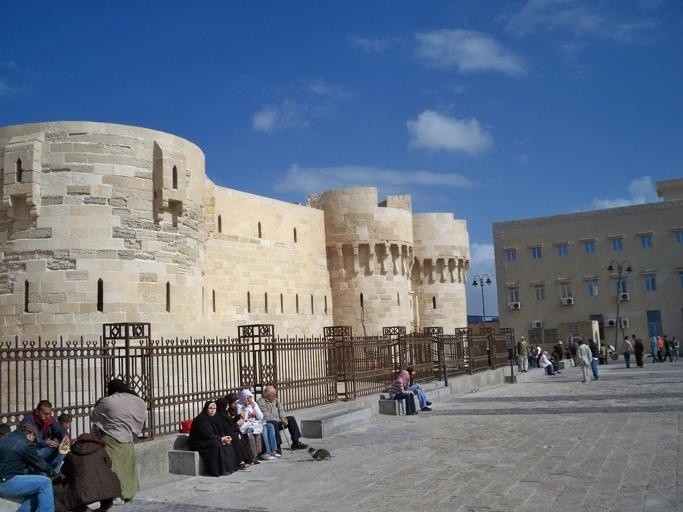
[{"left": 307, "top": 446, "right": 332, "bottom": 462}]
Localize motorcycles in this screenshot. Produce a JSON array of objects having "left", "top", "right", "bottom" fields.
[{"left": 607, "top": 343, "right": 618, "bottom": 361}]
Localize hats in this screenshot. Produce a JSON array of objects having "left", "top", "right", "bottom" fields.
[{"left": 19, "top": 423, "right": 38, "bottom": 443}]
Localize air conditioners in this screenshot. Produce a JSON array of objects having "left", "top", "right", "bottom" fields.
[
  {"left": 605, "top": 319, "right": 616, "bottom": 327},
  {"left": 618, "top": 319, "right": 629, "bottom": 328},
  {"left": 571, "top": 337, "right": 582, "bottom": 344},
  {"left": 561, "top": 298, "right": 574, "bottom": 305},
  {"left": 532, "top": 321, "right": 542, "bottom": 328},
  {"left": 509, "top": 302, "right": 520, "bottom": 309},
  {"left": 618, "top": 293, "right": 629, "bottom": 301}
]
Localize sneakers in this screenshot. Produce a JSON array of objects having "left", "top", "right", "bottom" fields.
[
  {"left": 205, "top": 441, "right": 312, "bottom": 476},
  {"left": 404, "top": 400, "right": 432, "bottom": 415}
]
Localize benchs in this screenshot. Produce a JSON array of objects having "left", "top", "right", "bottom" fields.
[
  {"left": 505, "top": 368, "right": 548, "bottom": 383},
  {"left": 301, "top": 407, "right": 371, "bottom": 439},
  {"left": 0, "top": 497, "right": 26, "bottom": 512},
  {"left": 379, "top": 387, "right": 450, "bottom": 416},
  {"left": 559, "top": 358, "right": 575, "bottom": 369},
  {"left": 169, "top": 446, "right": 207, "bottom": 476}
]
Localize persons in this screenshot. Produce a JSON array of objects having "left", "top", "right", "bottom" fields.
[
  {"left": 0, "top": 422, "right": 55, "bottom": 512},
  {"left": 389, "top": 370, "right": 419, "bottom": 416},
  {"left": 515, "top": 333, "right": 682, "bottom": 383},
  {"left": 89, "top": 378, "right": 149, "bottom": 505},
  {"left": 0, "top": 423, "right": 11, "bottom": 436},
  {"left": 19, "top": 399, "right": 71, "bottom": 474},
  {"left": 47, "top": 414, "right": 73, "bottom": 474},
  {"left": 405, "top": 367, "right": 433, "bottom": 411},
  {"left": 187, "top": 385, "right": 309, "bottom": 477},
  {"left": 52, "top": 432, "right": 123, "bottom": 512}
]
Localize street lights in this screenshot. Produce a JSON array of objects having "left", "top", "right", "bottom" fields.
[
  {"left": 472, "top": 272, "right": 493, "bottom": 334},
  {"left": 608, "top": 259, "right": 632, "bottom": 353}
]
